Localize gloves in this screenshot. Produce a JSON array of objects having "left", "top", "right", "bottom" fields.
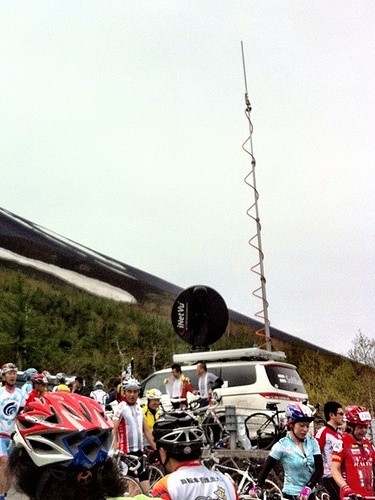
[{"left": 341, "top": 485, "right": 354, "bottom": 497}]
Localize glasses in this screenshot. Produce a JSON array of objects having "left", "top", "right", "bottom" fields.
[{"left": 337, "top": 412, "right": 344, "bottom": 416}]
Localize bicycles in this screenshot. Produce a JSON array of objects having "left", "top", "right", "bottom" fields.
[
  {"left": 244, "top": 399, "right": 309, "bottom": 450},
  {"left": 126, "top": 391, "right": 329, "bottom": 500}
]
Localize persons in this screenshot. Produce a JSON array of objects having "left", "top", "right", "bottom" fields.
[
  {"left": 152, "top": 409, "right": 238, "bottom": 500},
  {"left": 330, "top": 405, "right": 375, "bottom": 500},
  {"left": 0, "top": 362, "right": 195, "bottom": 500},
  {"left": 197, "top": 362, "right": 224, "bottom": 450},
  {"left": 254, "top": 404, "right": 324, "bottom": 500},
  {"left": 314, "top": 401, "right": 344, "bottom": 500}
]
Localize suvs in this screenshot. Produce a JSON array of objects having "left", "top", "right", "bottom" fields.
[{"left": 106, "top": 348, "right": 316, "bottom": 440}]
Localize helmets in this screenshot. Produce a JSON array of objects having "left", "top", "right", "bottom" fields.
[
  {"left": 42, "top": 371, "right": 51, "bottom": 379},
  {"left": 122, "top": 379, "right": 141, "bottom": 390},
  {"left": 56, "top": 373, "right": 66, "bottom": 379},
  {"left": 30, "top": 373, "right": 49, "bottom": 384},
  {"left": 12, "top": 390, "right": 116, "bottom": 468},
  {"left": 145, "top": 388, "right": 163, "bottom": 400},
  {"left": 344, "top": 404, "right": 372, "bottom": 425},
  {"left": 1, "top": 363, "right": 18, "bottom": 376},
  {"left": 22, "top": 368, "right": 38, "bottom": 383},
  {"left": 151, "top": 409, "right": 204, "bottom": 446},
  {"left": 95, "top": 380, "right": 104, "bottom": 388},
  {"left": 285, "top": 402, "right": 315, "bottom": 422}
]
